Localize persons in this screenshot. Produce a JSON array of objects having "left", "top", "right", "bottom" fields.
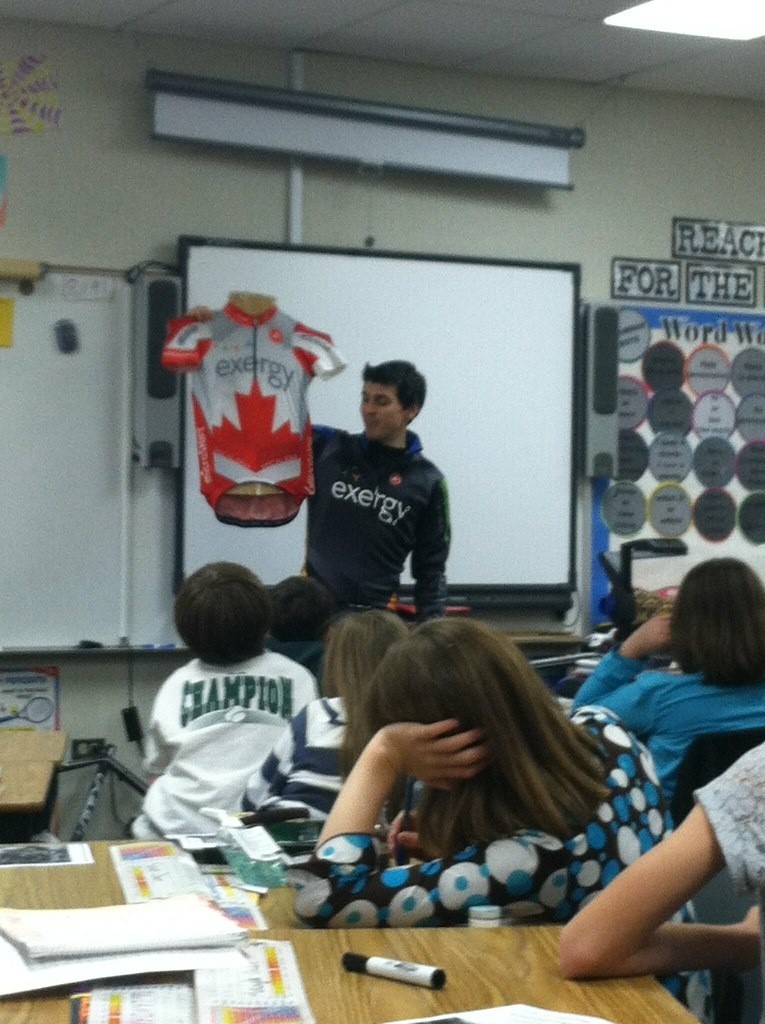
[
  {"left": 235, "top": 608, "right": 411, "bottom": 841},
  {"left": 562, "top": 740, "right": 765, "bottom": 980},
  {"left": 292, "top": 620, "right": 713, "bottom": 1024},
  {"left": 126, "top": 561, "right": 338, "bottom": 840},
  {"left": 302, "top": 360, "right": 450, "bottom": 616},
  {"left": 570, "top": 557, "right": 765, "bottom": 806}
]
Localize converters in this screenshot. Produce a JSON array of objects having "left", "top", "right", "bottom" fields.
[{"left": 122, "top": 705, "right": 144, "bottom": 742}]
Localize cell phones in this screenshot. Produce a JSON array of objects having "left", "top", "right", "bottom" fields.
[{"left": 193, "top": 847, "right": 232, "bottom": 874}]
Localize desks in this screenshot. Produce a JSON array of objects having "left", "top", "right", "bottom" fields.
[
  {"left": 505, "top": 630, "right": 583, "bottom": 661},
  {"left": 0, "top": 837, "right": 701, "bottom": 1023},
  {"left": 0, "top": 730, "right": 70, "bottom": 813}
]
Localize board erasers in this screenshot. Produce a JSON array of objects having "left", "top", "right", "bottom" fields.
[
  {"left": 55, "top": 317, "right": 80, "bottom": 354},
  {"left": 78, "top": 640, "right": 104, "bottom": 650}
]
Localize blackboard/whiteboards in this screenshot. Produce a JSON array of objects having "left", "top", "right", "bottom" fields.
[{"left": 0, "top": 254, "right": 194, "bottom": 662}]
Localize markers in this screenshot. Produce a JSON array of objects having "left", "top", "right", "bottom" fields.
[
  {"left": 142, "top": 642, "right": 188, "bottom": 649},
  {"left": 342, "top": 951, "right": 446, "bottom": 990}
]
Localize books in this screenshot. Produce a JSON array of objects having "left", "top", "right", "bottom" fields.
[{"left": 0, "top": 891, "right": 246, "bottom": 959}]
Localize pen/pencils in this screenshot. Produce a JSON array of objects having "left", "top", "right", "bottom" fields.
[{"left": 395, "top": 775, "right": 415, "bottom": 864}]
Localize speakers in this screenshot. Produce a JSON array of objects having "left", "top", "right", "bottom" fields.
[
  {"left": 582, "top": 304, "right": 621, "bottom": 479},
  {"left": 136, "top": 275, "right": 182, "bottom": 469}
]
